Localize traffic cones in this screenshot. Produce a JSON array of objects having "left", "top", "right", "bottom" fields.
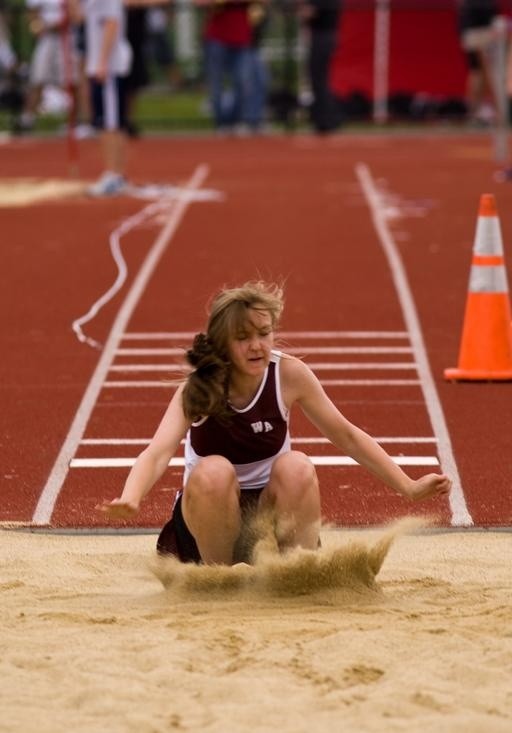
[{"left": 442, "top": 192, "right": 512, "bottom": 381}]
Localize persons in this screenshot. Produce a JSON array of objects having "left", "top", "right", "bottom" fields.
[
  {"left": 94, "top": 274, "right": 454, "bottom": 572},
  {"left": 1, "top": 0, "right": 512, "bottom": 136},
  {"left": 76, "top": 0, "right": 136, "bottom": 200}
]
